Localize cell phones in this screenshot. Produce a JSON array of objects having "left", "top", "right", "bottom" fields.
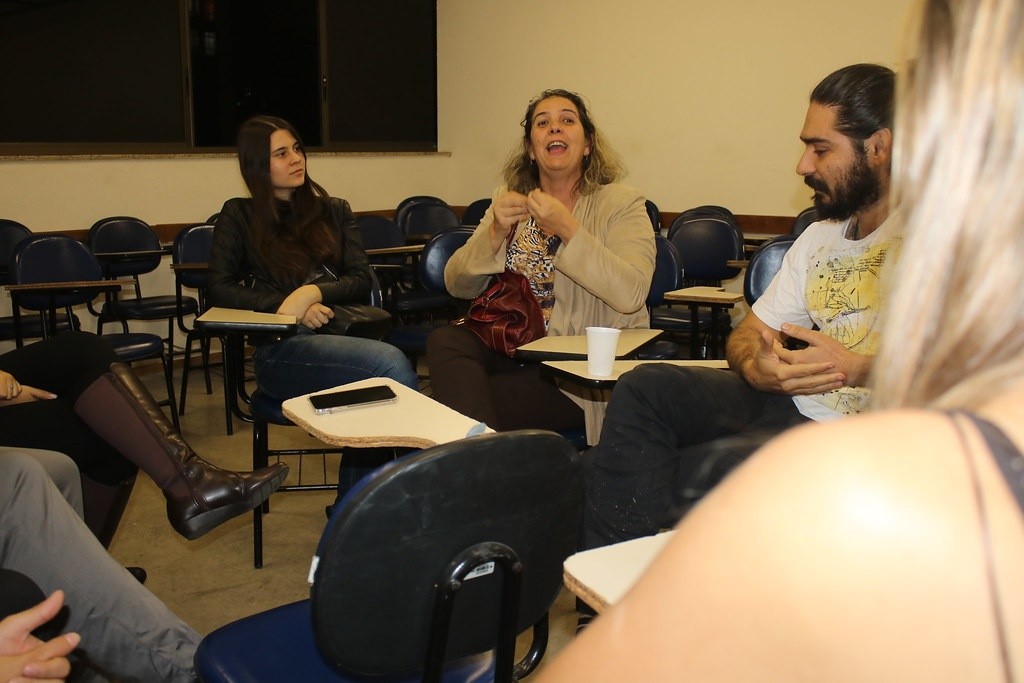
[{"left": 307, "top": 383, "right": 398, "bottom": 415}]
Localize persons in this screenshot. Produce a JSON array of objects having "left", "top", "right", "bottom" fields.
[
  {"left": 203, "top": 113, "right": 421, "bottom": 521},
  {"left": 0, "top": 331, "right": 290, "bottom": 683},
  {"left": 530, "top": 0, "right": 1024, "bottom": 682},
  {"left": 425, "top": 90, "right": 656, "bottom": 448}
]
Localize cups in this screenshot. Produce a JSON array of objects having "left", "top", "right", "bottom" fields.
[{"left": 586, "top": 325, "right": 622, "bottom": 376}]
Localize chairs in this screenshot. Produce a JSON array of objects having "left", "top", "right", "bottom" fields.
[{"left": 0, "top": 195, "right": 818, "bottom": 683}]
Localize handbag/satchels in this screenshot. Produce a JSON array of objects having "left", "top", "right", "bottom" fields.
[
  {"left": 447, "top": 221, "right": 547, "bottom": 358},
  {"left": 312, "top": 274, "right": 393, "bottom": 339}
]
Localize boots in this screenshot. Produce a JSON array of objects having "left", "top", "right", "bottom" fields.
[
  {"left": 77, "top": 471, "right": 147, "bottom": 586},
  {"left": 76, "top": 361, "right": 290, "bottom": 541}
]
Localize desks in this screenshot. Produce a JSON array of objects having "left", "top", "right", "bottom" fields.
[
  {"left": 279, "top": 377, "right": 498, "bottom": 452},
  {"left": 195, "top": 306, "right": 297, "bottom": 335},
  {"left": 516, "top": 329, "right": 665, "bottom": 360},
  {"left": 538, "top": 360, "right": 731, "bottom": 390},
  {"left": 169, "top": 262, "right": 207, "bottom": 273},
  {"left": 4, "top": 279, "right": 136, "bottom": 291},
  {"left": 93, "top": 249, "right": 171, "bottom": 257},
  {"left": 364, "top": 243, "right": 427, "bottom": 256},
  {"left": 662, "top": 286, "right": 745, "bottom": 309},
  {"left": 563, "top": 531, "right": 671, "bottom": 613}
]
